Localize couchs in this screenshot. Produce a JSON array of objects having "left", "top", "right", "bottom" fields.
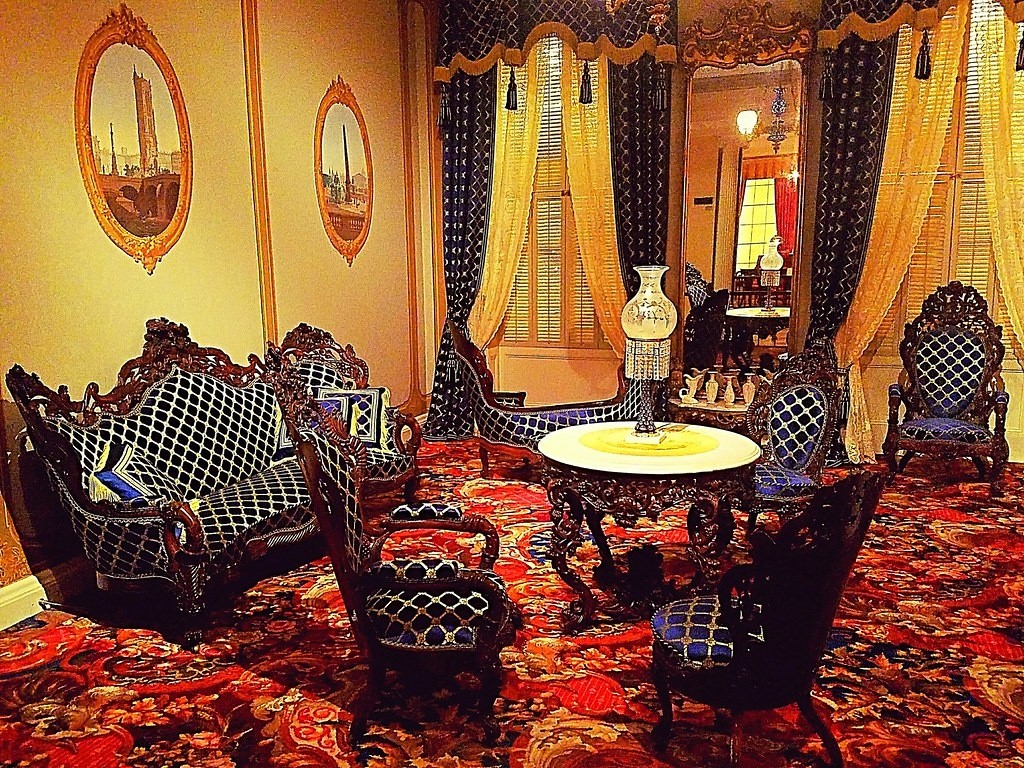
[{"left": 5, "top": 317, "right": 423, "bottom": 654}]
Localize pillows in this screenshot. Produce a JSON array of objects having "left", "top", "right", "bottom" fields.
[
  {"left": 271, "top": 397, "right": 358, "bottom": 468},
  {"left": 89, "top": 442, "right": 205, "bottom": 544},
  {"left": 318, "top": 386, "right": 396, "bottom": 455}
]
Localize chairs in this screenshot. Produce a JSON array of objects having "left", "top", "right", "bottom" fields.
[
  {"left": 686, "top": 260, "right": 732, "bottom": 340},
  {"left": 683, "top": 289, "right": 729, "bottom": 390},
  {"left": 882, "top": 281, "right": 1010, "bottom": 498},
  {"left": 731, "top": 337, "right": 855, "bottom": 540},
  {"left": 651, "top": 471, "right": 885, "bottom": 768},
  {"left": 265, "top": 339, "right": 517, "bottom": 741}
]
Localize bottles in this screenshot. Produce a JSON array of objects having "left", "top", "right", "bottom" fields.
[{"left": 704, "top": 363, "right": 762, "bottom": 408}]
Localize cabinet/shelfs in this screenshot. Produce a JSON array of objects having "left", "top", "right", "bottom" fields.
[{"left": 729, "top": 255, "right": 791, "bottom": 309}]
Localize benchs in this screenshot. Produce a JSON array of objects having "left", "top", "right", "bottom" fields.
[{"left": 446, "top": 320, "right": 640, "bottom": 480}]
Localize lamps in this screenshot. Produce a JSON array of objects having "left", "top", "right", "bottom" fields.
[
  {"left": 735, "top": 86, "right": 768, "bottom": 151},
  {"left": 757, "top": 236, "right": 784, "bottom": 316},
  {"left": 766, "top": 62, "right": 788, "bottom": 154},
  {"left": 621, "top": 266, "right": 677, "bottom": 445}
]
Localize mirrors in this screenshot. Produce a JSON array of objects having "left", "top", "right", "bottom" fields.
[{"left": 668, "top": 0, "right": 812, "bottom": 399}]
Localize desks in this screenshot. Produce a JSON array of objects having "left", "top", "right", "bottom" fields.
[
  {"left": 538, "top": 421, "right": 763, "bottom": 636},
  {"left": 719, "top": 307, "right": 790, "bottom": 374},
  {"left": 668, "top": 400, "right": 750, "bottom": 430}
]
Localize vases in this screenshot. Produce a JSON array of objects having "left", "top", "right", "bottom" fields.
[
  {"left": 713, "top": 365, "right": 724, "bottom": 395},
  {"left": 743, "top": 374, "right": 756, "bottom": 406},
  {"left": 706, "top": 371, "right": 719, "bottom": 404},
  {"left": 724, "top": 376, "right": 735, "bottom": 407},
  {"left": 729, "top": 369, "right": 741, "bottom": 394}
]
